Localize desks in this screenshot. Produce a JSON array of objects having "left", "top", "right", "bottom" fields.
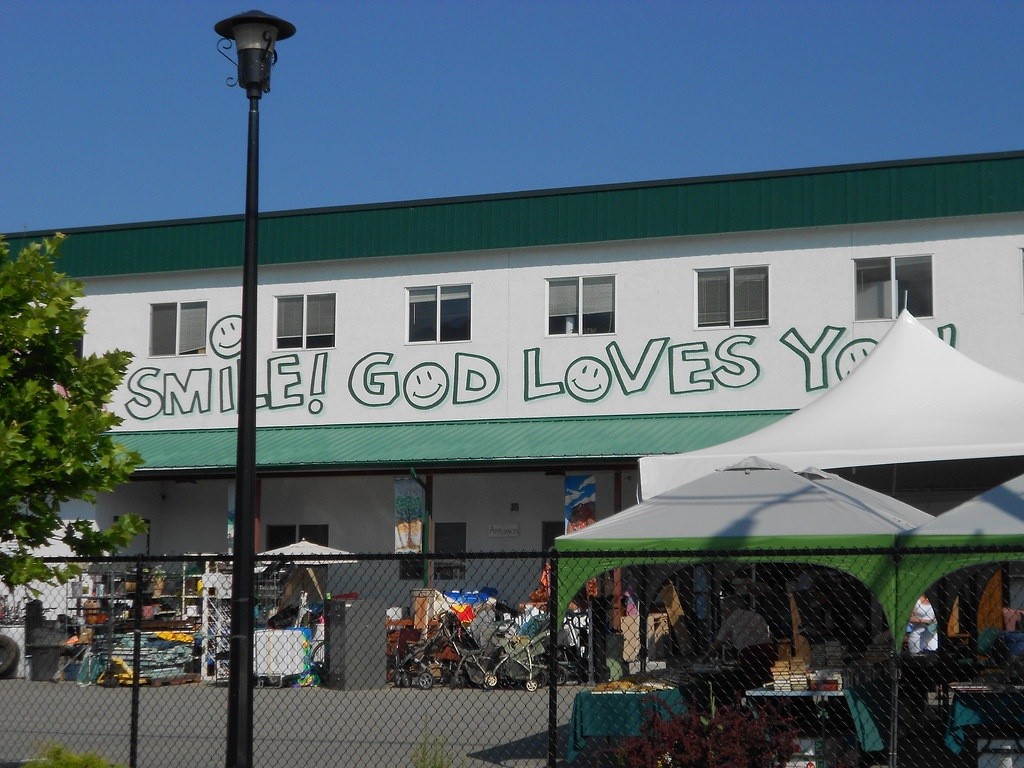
[
  {"left": 575, "top": 680, "right": 686, "bottom": 768},
  {"left": 747, "top": 686, "right": 854, "bottom": 768},
  {"left": 952, "top": 689, "right": 1024, "bottom": 726}
]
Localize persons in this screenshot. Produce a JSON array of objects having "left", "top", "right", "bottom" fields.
[
  {"left": 905, "top": 593, "right": 938, "bottom": 654},
  {"left": 698, "top": 590, "right": 773, "bottom": 716}
]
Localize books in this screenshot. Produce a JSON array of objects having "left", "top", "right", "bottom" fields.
[
  {"left": 587, "top": 659, "right": 740, "bottom": 694},
  {"left": 771, "top": 638, "right": 848, "bottom": 691}
]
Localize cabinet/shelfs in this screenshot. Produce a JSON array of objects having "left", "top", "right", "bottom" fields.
[{"left": 62, "top": 553, "right": 288, "bottom": 688}]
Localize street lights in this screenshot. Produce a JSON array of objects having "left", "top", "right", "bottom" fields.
[{"left": 209, "top": 8, "right": 301, "bottom": 768}]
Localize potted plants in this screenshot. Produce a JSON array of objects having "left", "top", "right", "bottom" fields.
[{"left": 147, "top": 565, "right": 166, "bottom": 597}]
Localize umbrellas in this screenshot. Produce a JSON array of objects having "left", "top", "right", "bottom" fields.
[{"left": 256, "top": 537, "right": 359, "bottom": 563}]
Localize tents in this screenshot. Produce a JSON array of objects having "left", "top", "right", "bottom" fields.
[
  {"left": 894, "top": 476, "right": 1024, "bottom": 768},
  {"left": 795, "top": 466, "right": 936, "bottom": 532},
  {"left": 638, "top": 309, "right": 1024, "bottom": 501},
  {"left": 548, "top": 457, "right": 912, "bottom": 768}
]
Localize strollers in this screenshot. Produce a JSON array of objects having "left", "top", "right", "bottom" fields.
[{"left": 380, "top": 607, "right": 607, "bottom": 692}]
